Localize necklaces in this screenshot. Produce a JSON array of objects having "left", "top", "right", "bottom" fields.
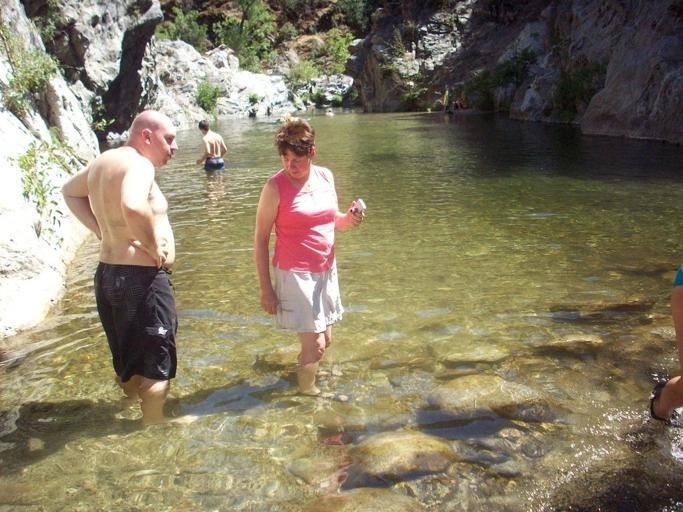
[{"left": 298, "top": 176, "right": 313, "bottom": 197}]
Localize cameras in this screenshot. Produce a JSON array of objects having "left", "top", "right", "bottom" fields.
[{"left": 349, "top": 198, "right": 367, "bottom": 215}]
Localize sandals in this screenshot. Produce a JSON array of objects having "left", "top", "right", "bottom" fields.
[{"left": 295, "top": 388, "right": 349, "bottom": 403}]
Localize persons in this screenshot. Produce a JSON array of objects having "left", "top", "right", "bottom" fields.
[
  {"left": 204, "top": 174, "right": 226, "bottom": 236},
  {"left": 640, "top": 256, "right": 682, "bottom": 443},
  {"left": 323, "top": 110, "right": 334, "bottom": 118},
  {"left": 454, "top": 90, "right": 469, "bottom": 110},
  {"left": 441, "top": 84, "right": 451, "bottom": 112},
  {"left": 194, "top": 118, "right": 227, "bottom": 173},
  {"left": 61, "top": 107, "right": 180, "bottom": 427},
  {"left": 252, "top": 115, "right": 370, "bottom": 404}
]
